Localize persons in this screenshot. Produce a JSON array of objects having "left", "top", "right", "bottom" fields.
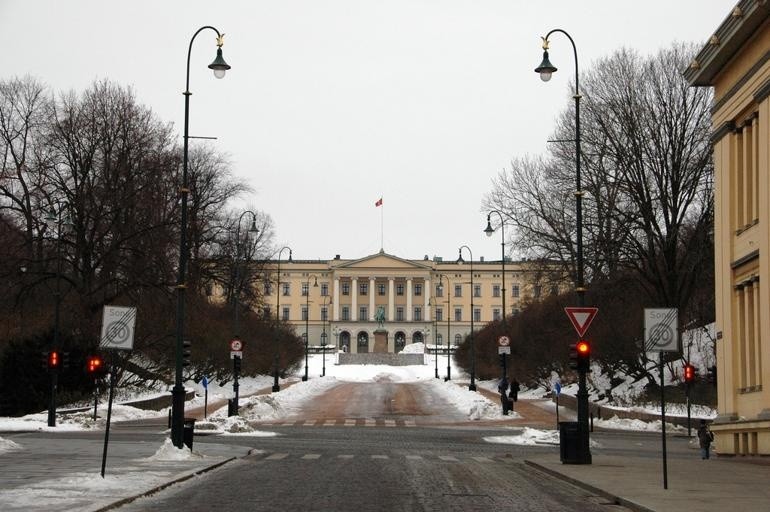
[
  {"left": 698, "top": 419, "right": 714, "bottom": 460},
  {"left": 508, "top": 378, "right": 520, "bottom": 402}
]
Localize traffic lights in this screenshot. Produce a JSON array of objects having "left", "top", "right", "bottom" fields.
[
  {"left": 87, "top": 356, "right": 103, "bottom": 380},
  {"left": 682, "top": 365, "right": 695, "bottom": 385},
  {"left": 49, "top": 350, "right": 63, "bottom": 371},
  {"left": 576, "top": 341, "right": 591, "bottom": 372}
]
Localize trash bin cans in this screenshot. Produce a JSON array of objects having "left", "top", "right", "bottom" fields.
[
  {"left": 556, "top": 421, "right": 581, "bottom": 466},
  {"left": 184, "top": 417, "right": 198, "bottom": 453}
]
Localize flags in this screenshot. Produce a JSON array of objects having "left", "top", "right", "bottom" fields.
[{"left": 375, "top": 198, "right": 382, "bottom": 207}]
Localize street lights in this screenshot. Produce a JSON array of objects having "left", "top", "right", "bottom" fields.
[
  {"left": 234, "top": 210, "right": 333, "bottom": 404},
  {"left": 534, "top": 29, "right": 588, "bottom": 422},
  {"left": 483, "top": 210, "right": 509, "bottom": 415},
  {"left": 43, "top": 196, "right": 61, "bottom": 427},
  {"left": 170, "top": 25, "right": 231, "bottom": 447},
  {"left": 428, "top": 245, "right": 477, "bottom": 390}
]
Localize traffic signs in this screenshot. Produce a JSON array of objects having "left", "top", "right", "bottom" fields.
[
  {"left": 643, "top": 307, "right": 679, "bottom": 352},
  {"left": 99, "top": 305, "right": 137, "bottom": 351}
]
